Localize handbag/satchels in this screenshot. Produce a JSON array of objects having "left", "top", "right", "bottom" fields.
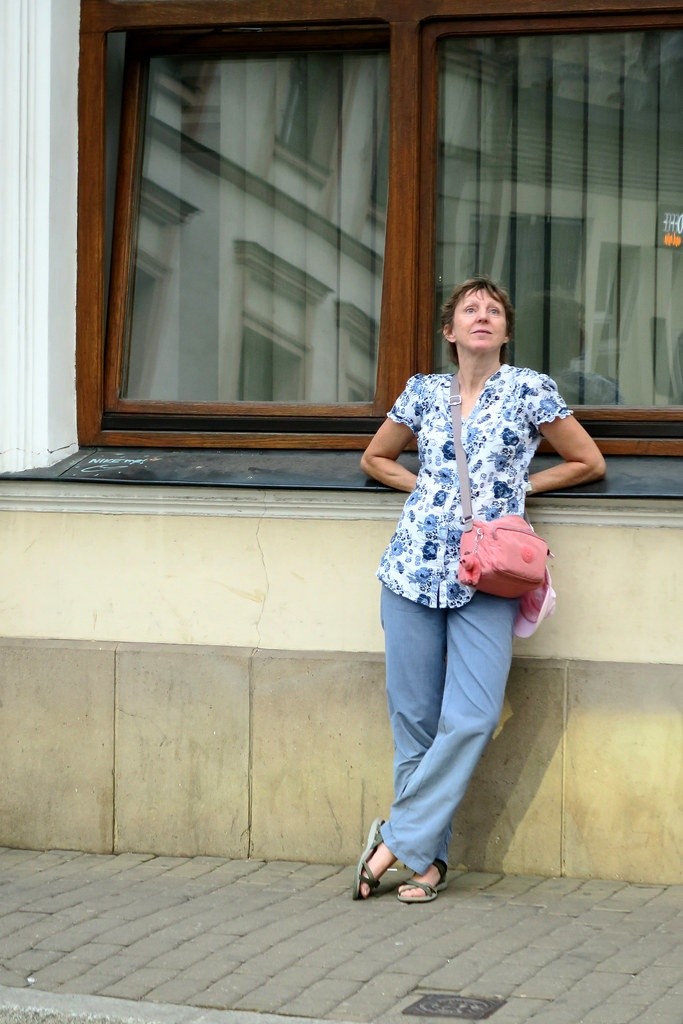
[{"left": 457, "top": 516, "right": 554, "bottom": 598}]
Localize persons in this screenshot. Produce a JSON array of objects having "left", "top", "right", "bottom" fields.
[{"left": 352, "top": 278, "right": 608, "bottom": 903}]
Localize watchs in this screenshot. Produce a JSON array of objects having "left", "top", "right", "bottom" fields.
[{"left": 526, "top": 481, "right": 533, "bottom": 495}]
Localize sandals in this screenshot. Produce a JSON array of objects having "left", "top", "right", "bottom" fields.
[
  {"left": 397, "top": 859, "right": 447, "bottom": 902},
  {"left": 352, "top": 820, "right": 385, "bottom": 900}
]
